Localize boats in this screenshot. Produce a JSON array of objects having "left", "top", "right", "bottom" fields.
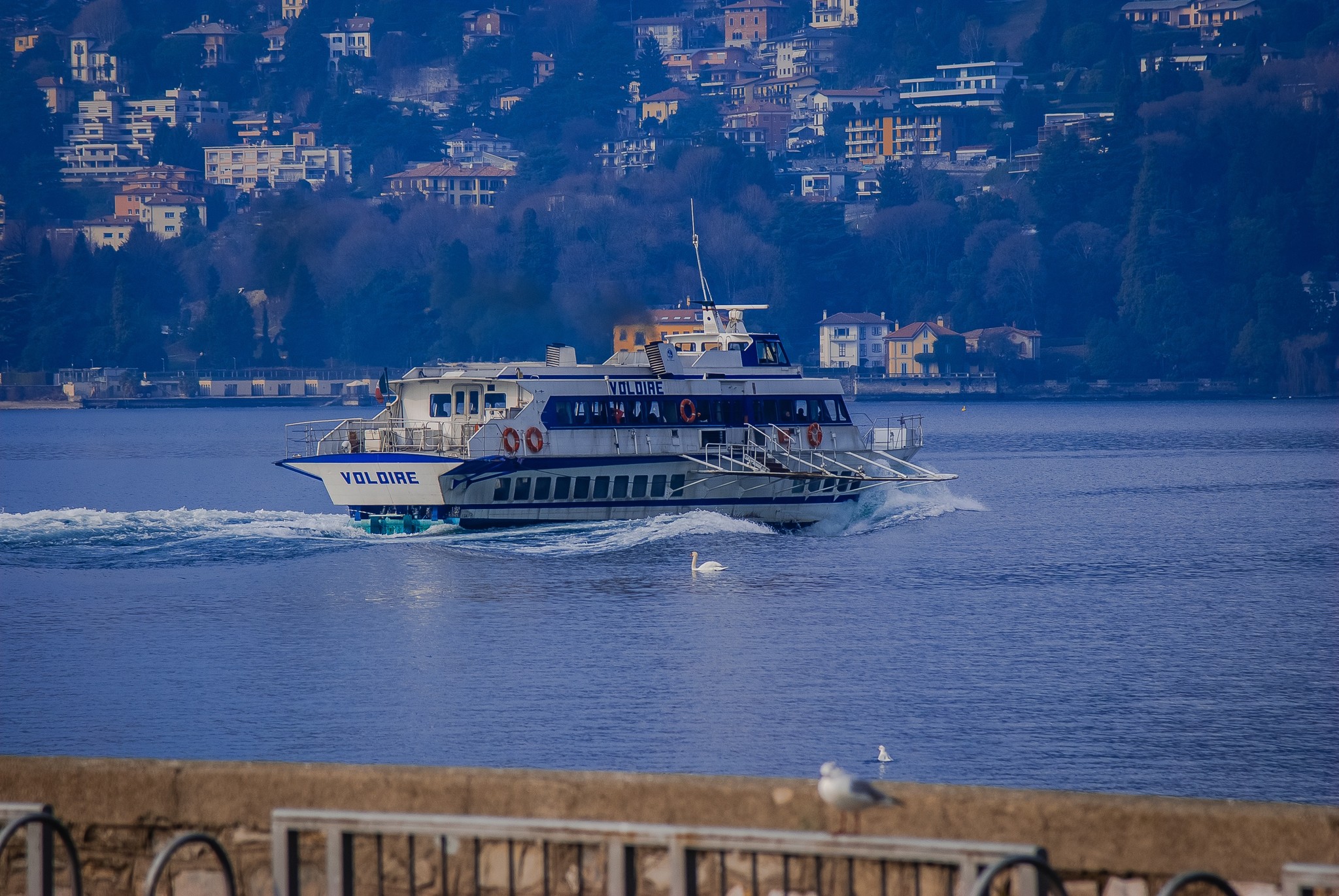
[{"left": 270, "top": 199, "right": 960, "bottom": 536}]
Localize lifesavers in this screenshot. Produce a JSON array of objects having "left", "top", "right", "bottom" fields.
[
  {"left": 526, "top": 427, "right": 543, "bottom": 452},
  {"left": 808, "top": 422, "right": 823, "bottom": 447},
  {"left": 680, "top": 399, "right": 696, "bottom": 423},
  {"left": 503, "top": 427, "right": 520, "bottom": 452}
]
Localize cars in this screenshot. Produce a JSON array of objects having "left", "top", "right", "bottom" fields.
[
  {"left": 774, "top": 167, "right": 813, "bottom": 172},
  {"left": 863, "top": 165, "right": 876, "bottom": 171}
]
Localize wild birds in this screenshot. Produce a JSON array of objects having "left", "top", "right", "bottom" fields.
[
  {"left": 688, "top": 551, "right": 730, "bottom": 572},
  {"left": 237, "top": 287, "right": 246, "bottom": 294},
  {"left": 1289, "top": 396, "right": 1292, "bottom": 398},
  {"left": 816, "top": 760, "right": 909, "bottom": 841},
  {"left": 961, "top": 405, "right": 966, "bottom": 412},
  {"left": 1271, "top": 396, "right": 1277, "bottom": 400},
  {"left": 877, "top": 744, "right": 894, "bottom": 763},
  {"left": 472, "top": 122, "right": 475, "bottom": 127}
]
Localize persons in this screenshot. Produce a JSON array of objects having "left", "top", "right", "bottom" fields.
[
  {"left": 796, "top": 408, "right": 807, "bottom": 421},
  {"left": 600, "top": 407, "right": 659, "bottom": 423},
  {"left": 818, "top": 410, "right": 831, "bottom": 422}
]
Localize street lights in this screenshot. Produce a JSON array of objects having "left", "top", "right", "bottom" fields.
[
  {"left": 1006, "top": 134, "right": 1011, "bottom": 163},
  {"left": 1034, "top": 332, "right": 1037, "bottom": 361},
  {"left": 90, "top": 359, "right": 93, "bottom": 369},
  {"left": 233, "top": 357, "right": 236, "bottom": 371},
  {"left": 6, "top": 360, "right": 9, "bottom": 372},
  {"left": 161, "top": 358, "right": 165, "bottom": 373}
]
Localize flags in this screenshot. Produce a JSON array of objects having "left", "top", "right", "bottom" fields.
[{"left": 375, "top": 373, "right": 387, "bottom": 404}]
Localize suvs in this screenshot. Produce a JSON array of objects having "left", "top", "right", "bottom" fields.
[{"left": 971, "top": 154, "right": 986, "bottom": 161}]
